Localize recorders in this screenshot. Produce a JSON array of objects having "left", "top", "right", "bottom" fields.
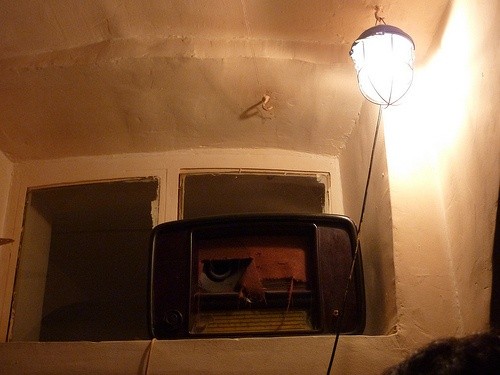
[{"left": 147, "top": 213, "right": 368, "bottom": 343}]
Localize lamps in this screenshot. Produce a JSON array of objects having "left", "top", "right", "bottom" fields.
[{"left": 348, "top": 7, "right": 416, "bottom": 109}]
